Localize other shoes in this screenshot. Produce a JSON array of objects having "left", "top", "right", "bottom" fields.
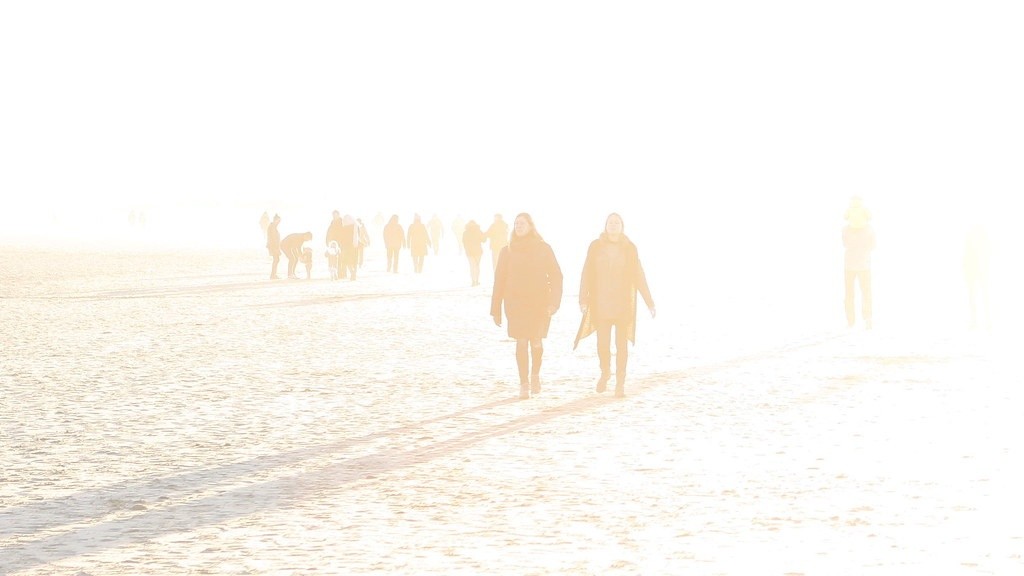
[
  {"left": 597, "top": 371, "right": 611, "bottom": 393},
  {"left": 531, "top": 376, "right": 541, "bottom": 394},
  {"left": 614, "top": 384, "right": 625, "bottom": 398},
  {"left": 519, "top": 379, "right": 529, "bottom": 398}
]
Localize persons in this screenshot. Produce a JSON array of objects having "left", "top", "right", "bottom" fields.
[
  {"left": 464, "top": 220, "right": 485, "bottom": 284},
  {"left": 491, "top": 214, "right": 563, "bottom": 397},
  {"left": 574, "top": 214, "right": 656, "bottom": 397},
  {"left": 258, "top": 208, "right": 469, "bottom": 283},
  {"left": 484, "top": 218, "right": 510, "bottom": 263},
  {"left": 842, "top": 201, "right": 878, "bottom": 322}
]
